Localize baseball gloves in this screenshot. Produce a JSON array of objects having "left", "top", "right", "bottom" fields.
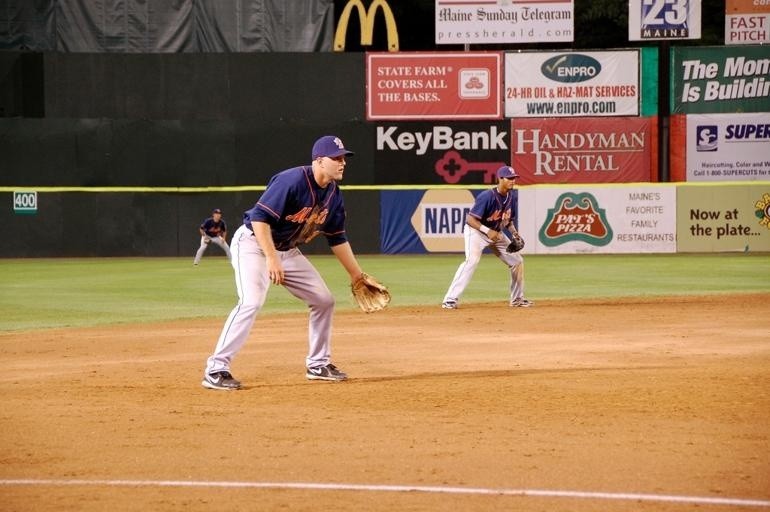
[
  {"left": 506, "top": 237, "right": 524, "bottom": 253},
  {"left": 352, "top": 272, "right": 390, "bottom": 313},
  {"left": 204, "top": 237, "right": 211, "bottom": 242}
]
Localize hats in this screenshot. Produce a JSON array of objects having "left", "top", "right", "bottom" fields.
[
  {"left": 311, "top": 136, "right": 355, "bottom": 161},
  {"left": 213, "top": 208, "right": 222, "bottom": 214},
  {"left": 497, "top": 166, "right": 520, "bottom": 178}
]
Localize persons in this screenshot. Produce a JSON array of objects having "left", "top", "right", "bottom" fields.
[
  {"left": 194, "top": 209, "right": 231, "bottom": 265},
  {"left": 203, "top": 135, "right": 393, "bottom": 390},
  {"left": 442, "top": 166, "right": 534, "bottom": 310}
]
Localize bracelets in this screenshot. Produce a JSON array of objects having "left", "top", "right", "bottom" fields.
[{"left": 480, "top": 224, "right": 490, "bottom": 235}]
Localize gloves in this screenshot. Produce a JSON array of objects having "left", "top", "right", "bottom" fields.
[{"left": 487, "top": 229, "right": 501, "bottom": 242}]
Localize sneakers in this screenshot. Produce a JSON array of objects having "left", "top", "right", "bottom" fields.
[
  {"left": 201, "top": 372, "right": 241, "bottom": 390},
  {"left": 509, "top": 298, "right": 534, "bottom": 307},
  {"left": 306, "top": 364, "right": 347, "bottom": 381},
  {"left": 441, "top": 302, "right": 457, "bottom": 309}
]
